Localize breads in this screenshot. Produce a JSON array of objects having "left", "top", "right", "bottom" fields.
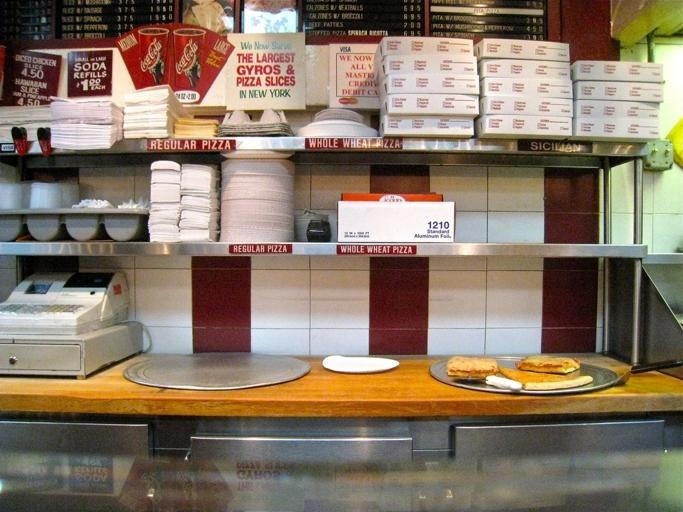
[
  {"left": 514, "top": 354, "right": 581, "bottom": 374},
  {"left": 499, "top": 362, "right": 593, "bottom": 391},
  {"left": 446, "top": 355, "right": 499, "bottom": 378}
]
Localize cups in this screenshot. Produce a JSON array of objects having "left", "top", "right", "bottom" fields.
[
  {"left": 171, "top": 29, "right": 205, "bottom": 89},
  {"left": 293, "top": 214, "right": 328, "bottom": 242},
  {"left": 0, "top": 180, "right": 79, "bottom": 212},
  {"left": 137, "top": 27, "right": 169, "bottom": 89}
]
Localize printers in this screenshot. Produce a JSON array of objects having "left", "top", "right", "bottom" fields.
[{"left": 0, "top": 270, "right": 129, "bottom": 334}]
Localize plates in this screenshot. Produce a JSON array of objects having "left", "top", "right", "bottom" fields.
[{"left": 322, "top": 354, "right": 400, "bottom": 375}]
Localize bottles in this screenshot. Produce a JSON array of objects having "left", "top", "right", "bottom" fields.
[{"left": 306, "top": 219, "right": 331, "bottom": 242}]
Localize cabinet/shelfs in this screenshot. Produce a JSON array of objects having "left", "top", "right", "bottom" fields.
[{"left": 0, "top": 136, "right": 650, "bottom": 367}]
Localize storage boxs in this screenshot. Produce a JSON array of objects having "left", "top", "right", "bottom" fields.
[
  {"left": 337, "top": 193, "right": 455, "bottom": 243},
  {"left": 372, "top": 35, "right": 665, "bottom": 142}
]
